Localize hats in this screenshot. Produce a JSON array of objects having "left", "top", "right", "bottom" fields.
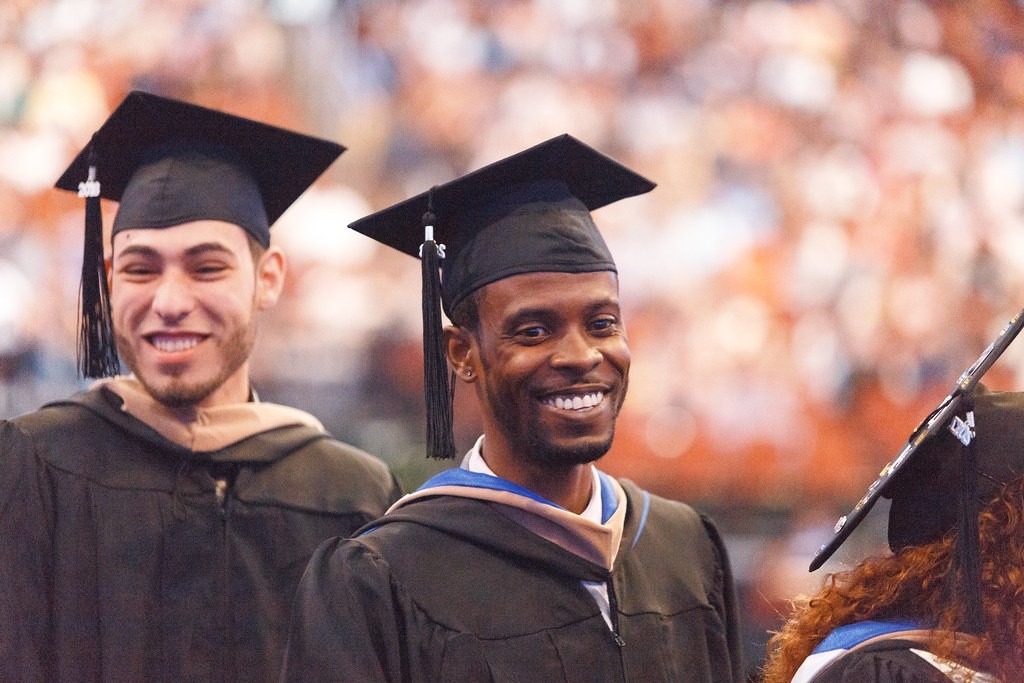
[
  {"left": 347, "top": 134, "right": 659, "bottom": 464},
  {"left": 807, "top": 308, "right": 1024, "bottom": 636},
  {"left": 53, "top": 90, "right": 349, "bottom": 385}
]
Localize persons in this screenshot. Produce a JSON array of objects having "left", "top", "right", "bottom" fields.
[
  {"left": 761, "top": 308, "right": 1024, "bottom": 683},
  {"left": 281, "top": 134, "right": 745, "bottom": 683},
  {"left": 0, "top": 89, "right": 403, "bottom": 683}
]
